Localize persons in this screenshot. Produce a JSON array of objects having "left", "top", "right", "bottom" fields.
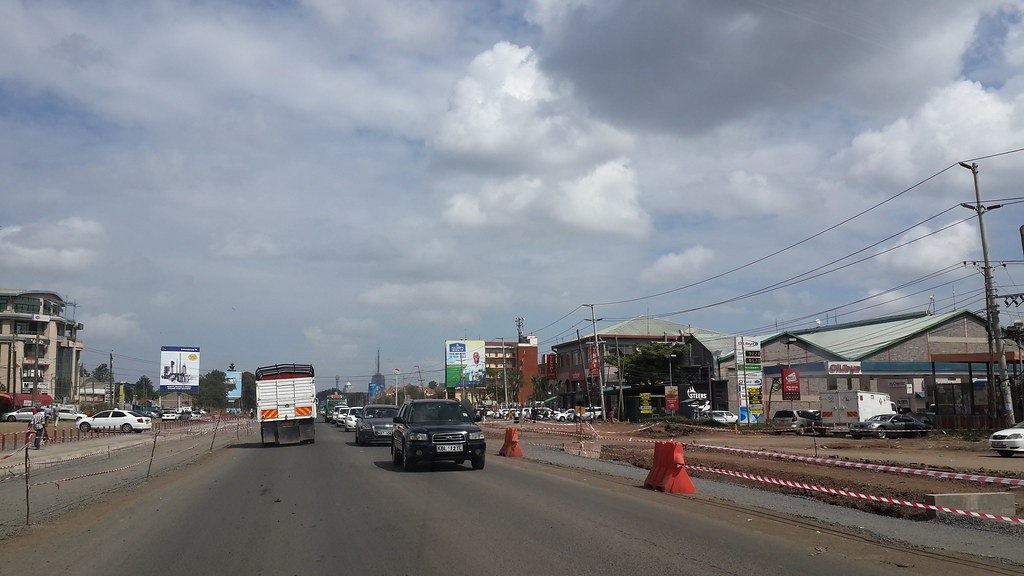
[
  {"left": 33, "top": 406, "right": 37, "bottom": 415},
  {"left": 44, "top": 406, "right": 51, "bottom": 424},
  {"left": 461, "top": 352, "right": 486, "bottom": 381},
  {"left": 54, "top": 405, "right": 60, "bottom": 427},
  {"left": 28, "top": 409, "right": 47, "bottom": 450}
]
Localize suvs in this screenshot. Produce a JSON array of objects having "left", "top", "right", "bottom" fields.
[
  {"left": 354, "top": 404, "right": 401, "bottom": 445},
  {"left": 773, "top": 409, "right": 825, "bottom": 436},
  {"left": 700, "top": 410, "right": 739, "bottom": 424},
  {"left": 391, "top": 397, "right": 486, "bottom": 472}
]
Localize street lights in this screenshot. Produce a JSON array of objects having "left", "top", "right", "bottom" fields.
[
  {"left": 669, "top": 354, "right": 677, "bottom": 416},
  {"left": 785, "top": 338, "right": 797, "bottom": 409}
]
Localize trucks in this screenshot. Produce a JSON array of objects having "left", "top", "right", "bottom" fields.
[
  {"left": 324, "top": 395, "right": 348, "bottom": 423},
  {"left": 256, "top": 362, "right": 319, "bottom": 447},
  {"left": 819, "top": 390, "right": 902, "bottom": 437}
]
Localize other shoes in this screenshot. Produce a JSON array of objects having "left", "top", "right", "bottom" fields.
[{"left": 35, "top": 443, "right": 40, "bottom": 447}]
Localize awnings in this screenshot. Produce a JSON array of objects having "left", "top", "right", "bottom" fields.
[{"left": 0, "top": 393, "right": 53, "bottom": 408}]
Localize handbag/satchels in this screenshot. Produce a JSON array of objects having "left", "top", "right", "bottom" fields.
[{"left": 42, "top": 430, "right": 49, "bottom": 441}]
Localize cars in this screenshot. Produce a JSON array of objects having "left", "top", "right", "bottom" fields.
[
  {"left": 140, "top": 406, "right": 209, "bottom": 423},
  {"left": 332, "top": 405, "right": 363, "bottom": 432},
  {"left": 478, "top": 404, "right": 602, "bottom": 422},
  {"left": 57, "top": 407, "right": 88, "bottom": 422},
  {"left": 336, "top": 408, "right": 349, "bottom": 427},
  {"left": 1, "top": 406, "right": 51, "bottom": 423},
  {"left": 989, "top": 420, "right": 1024, "bottom": 457},
  {"left": 850, "top": 413, "right": 929, "bottom": 440},
  {"left": 75, "top": 407, "right": 153, "bottom": 434}
]
